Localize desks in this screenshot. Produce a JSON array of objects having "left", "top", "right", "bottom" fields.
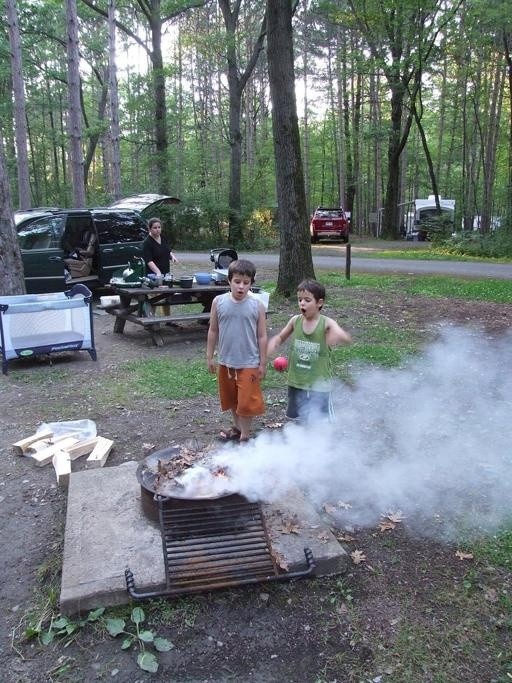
[{"left": 104, "top": 281, "right": 260, "bottom": 345}]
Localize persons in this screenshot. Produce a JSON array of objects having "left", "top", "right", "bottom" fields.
[
  {"left": 141, "top": 217, "right": 184, "bottom": 331},
  {"left": 267, "top": 278, "right": 352, "bottom": 431},
  {"left": 206, "top": 260, "right": 268, "bottom": 450}
]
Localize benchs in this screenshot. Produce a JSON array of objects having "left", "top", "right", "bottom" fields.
[
  {"left": 96, "top": 300, "right": 203, "bottom": 333},
  {"left": 135, "top": 310, "right": 274, "bottom": 346}
]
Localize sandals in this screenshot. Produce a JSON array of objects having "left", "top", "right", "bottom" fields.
[{"left": 218, "top": 426, "right": 249, "bottom": 442}]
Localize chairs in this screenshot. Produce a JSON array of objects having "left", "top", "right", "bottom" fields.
[{"left": 64, "top": 229, "right": 96, "bottom": 276}]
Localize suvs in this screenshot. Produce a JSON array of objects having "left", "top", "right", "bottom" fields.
[{"left": 310, "top": 208, "right": 349, "bottom": 243}]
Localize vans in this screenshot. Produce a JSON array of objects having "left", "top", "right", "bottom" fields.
[{"left": 12, "top": 193, "right": 183, "bottom": 294}]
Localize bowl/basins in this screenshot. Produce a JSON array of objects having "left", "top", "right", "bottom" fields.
[{"left": 194, "top": 272, "right": 211, "bottom": 284}]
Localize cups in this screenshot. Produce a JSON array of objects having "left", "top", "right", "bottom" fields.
[{"left": 167, "top": 273, "right": 174, "bottom": 288}]
